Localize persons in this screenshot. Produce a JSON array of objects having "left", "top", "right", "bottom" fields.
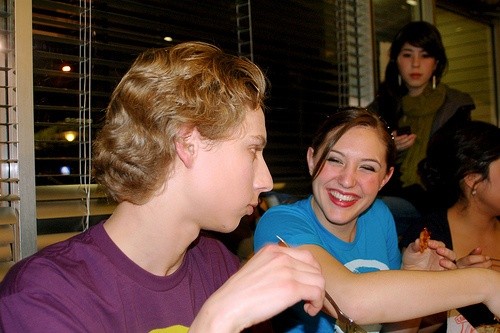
[
  {"left": 254, "top": 106, "right": 500, "bottom": 333},
  {"left": 398, "top": 117, "right": 500, "bottom": 333},
  {"left": 365, "top": 21, "right": 476, "bottom": 230},
  {"left": 0, "top": 43, "right": 325, "bottom": 333}
]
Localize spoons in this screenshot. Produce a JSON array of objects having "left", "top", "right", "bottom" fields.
[{"left": 276, "top": 235, "right": 367, "bottom": 333}]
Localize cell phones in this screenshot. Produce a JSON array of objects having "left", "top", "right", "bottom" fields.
[{"left": 397, "top": 126, "right": 412, "bottom": 136}]
